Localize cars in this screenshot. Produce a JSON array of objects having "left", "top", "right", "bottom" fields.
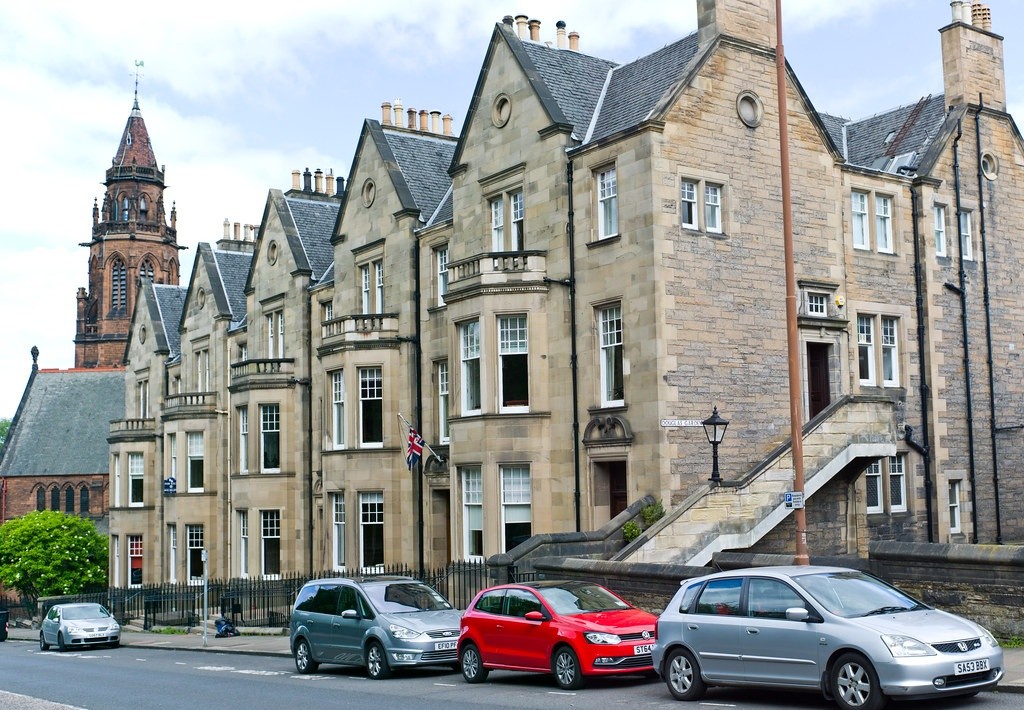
[
  {"left": 287, "top": 575, "right": 465, "bottom": 681},
  {"left": 39, "top": 603, "right": 121, "bottom": 653},
  {"left": 650, "top": 562, "right": 1005, "bottom": 710},
  {"left": 0, "top": 607, "right": 11, "bottom": 642}
]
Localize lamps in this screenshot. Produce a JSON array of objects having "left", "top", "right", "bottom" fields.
[{"left": 701, "top": 406, "right": 730, "bottom": 482}]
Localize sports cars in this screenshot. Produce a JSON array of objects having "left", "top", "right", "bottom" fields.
[{"left": 454, "top": 580, "right": 661, "bottom": 690}]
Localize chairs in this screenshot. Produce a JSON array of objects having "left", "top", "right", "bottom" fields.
[
  {"left": 221, "top": 597, "right": 244, "bottom": 622},
  {"left": 759, "top": 589, "right": 785, "bottom": 612},
  {"left": 268, "top": 610, "right": 290, "bottom": 627}
]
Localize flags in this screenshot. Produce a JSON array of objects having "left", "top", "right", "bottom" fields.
[{"left": 406, "top": 425, "right": 426, "bottom": 471}]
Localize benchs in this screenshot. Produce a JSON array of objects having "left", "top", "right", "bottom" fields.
[{"left": 701, "top": 590, "right": 740, "bottom": 612}]
